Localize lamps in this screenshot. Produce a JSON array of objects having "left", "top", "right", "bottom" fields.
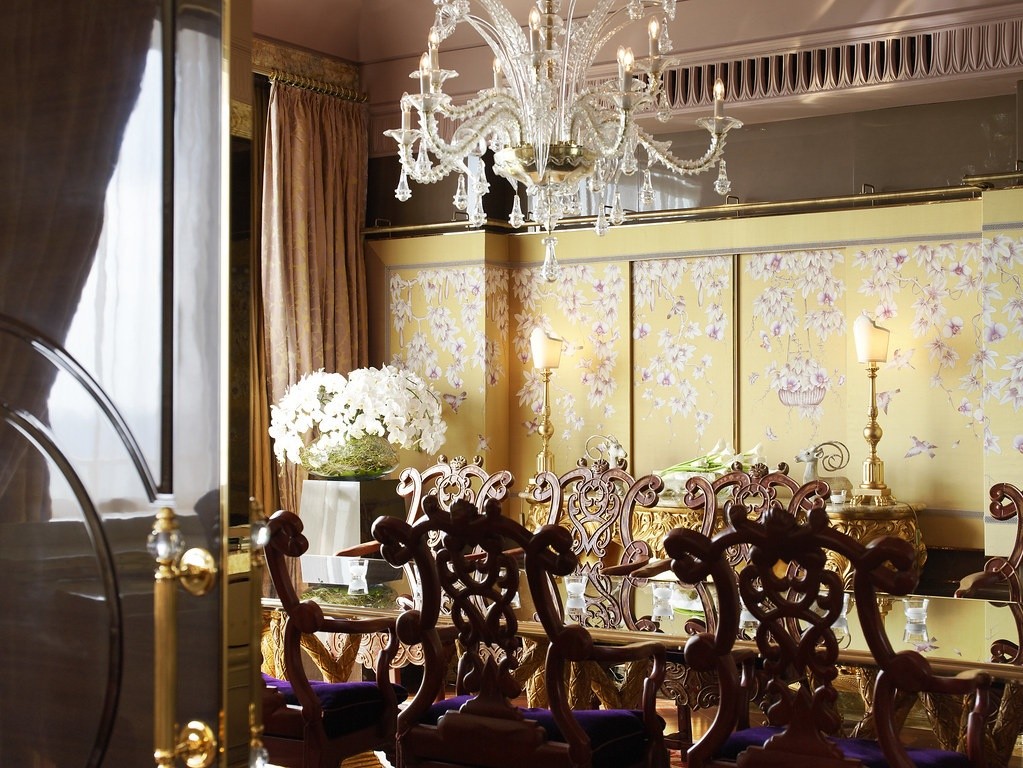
[
  {"left": 382, "top": 1, "right": 738, "bottom": 285},
  {"left": 847, "top": 314, "right": 900, "bottom": 507},
  {"left": 523, "top": 327, "right": 566, "bottom": 494}
]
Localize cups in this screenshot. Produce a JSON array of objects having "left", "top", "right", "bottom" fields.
[
  {"left": 829, "top": 616, "right": 849, "bottom": 638},
  {"left": 737, "top": 608, "right": 762, "bottom": 630},
  {"left": 346, "top": 559, "right": 369, "bottom": 580},
  {"left": 837, "top": 593, "right": 850, "bottom": 615},
  {"left": 347, "top": 579, "right": 370, "bottom": 596},
  {"left": 901, "top": 597, "right": 929, "bottom": 620},
  {"left": 564, "top": 575, "right": 589, "bottom": 597},
  {"left": 564, "top": 597, "right": 588, "bottom": 616},
  {"left": 651, "top": 581, "right": 676, "bottom": 603},
  {"left": 500, "top": 588, "right": 521, "bottom": 611},
  {"left": 652, "top": 603, "right": 676, "bottom": 622},
  {"left": 902, "top": 621, "right": 930, "bottom": 643},
  {"left": 830, "top": 489, "right": 848, "bottom": 504}
]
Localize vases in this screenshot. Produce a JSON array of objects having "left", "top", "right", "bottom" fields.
[{"left": 299, "top": 430, "right": 400, "bottom": 480}]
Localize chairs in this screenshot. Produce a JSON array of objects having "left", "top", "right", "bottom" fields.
[{"left": 260, "top": 452, "right": 1023, "bottom": 766}]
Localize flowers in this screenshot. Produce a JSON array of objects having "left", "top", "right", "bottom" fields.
[{"left": 267, "top": 362, "right": 448, "bottom": 465}]
